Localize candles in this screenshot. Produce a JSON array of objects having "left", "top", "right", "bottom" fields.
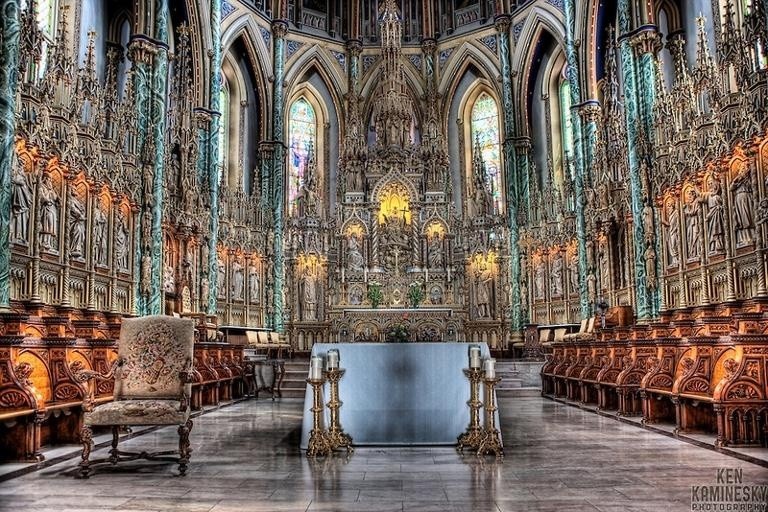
[
  {"left": 309, "top": 348, "right": 341, "bottom": 380},
  {"left": 468, "top": 344, "right": 497, "bottom": 382}
]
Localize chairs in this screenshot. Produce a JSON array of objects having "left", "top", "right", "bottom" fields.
[{"left": 74, "top": 314, "right": 197, "bottom": 480}]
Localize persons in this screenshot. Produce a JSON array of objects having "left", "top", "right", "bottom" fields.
[
  {"left": 428, "top": 230, "right": 445, "bottom": 269},
  {"left": 693, "top": 175, "right": 724, "bottom": 253},
  {"left": 661, "top": 197, "right": 681, "bottom": 268},
  {"left": 474, "top": 261, "right": 494, "bottom": 320},
  {"left": 232, "top": 255, "right": 244, "bottom": 304},
  {"left": 640, "top": 201, "right": 655, "bottom": 235},
  {"left": 93, "top": 186, "right": 108, "bottom": 264},
  {"left": 583, "top": 234, "right": 595, "bottom": 265},
  {"left": 37, "top": 165, "right": 62, "bottom": 254},
  {"left": 644, "top": 245, "right": 656, "bottom": 275},
  {"left": 8, "top": 148, "right": 33, "bottom": 243},
  {"left": 584, "top": 269, "right": 597, "bottom": 297},
  {"left": 386, "top": 205, "right": 406, "bottom": 229},
  {"left": 568, "top": 249, "right": 580, "bottom": 293},
  {"left": 249, "top": 257, "right": 261, "bottom": 306},
  {"left": 217, "top": 257, "right": 227, "bottom": 299},
  {"left": 345, "top": 231, "right": 365, "bottom": 269},
  {"left": 67, "top": 176, "right": 89, "bottom": 263},
  {"left": 681, "top": 189, "right": 706, "bottom": 259},
  {"left": 532, "top": 254, "right": 544, "bottom": 298},
  {"left": 113, "top": 196, "right": 131, "bottom": 270},
  {"left": 730, "top": 161, "right": 753, "bottom": 246},
  {"left": 548, "top": 250, "right": 562, "bottom": 297}
]
